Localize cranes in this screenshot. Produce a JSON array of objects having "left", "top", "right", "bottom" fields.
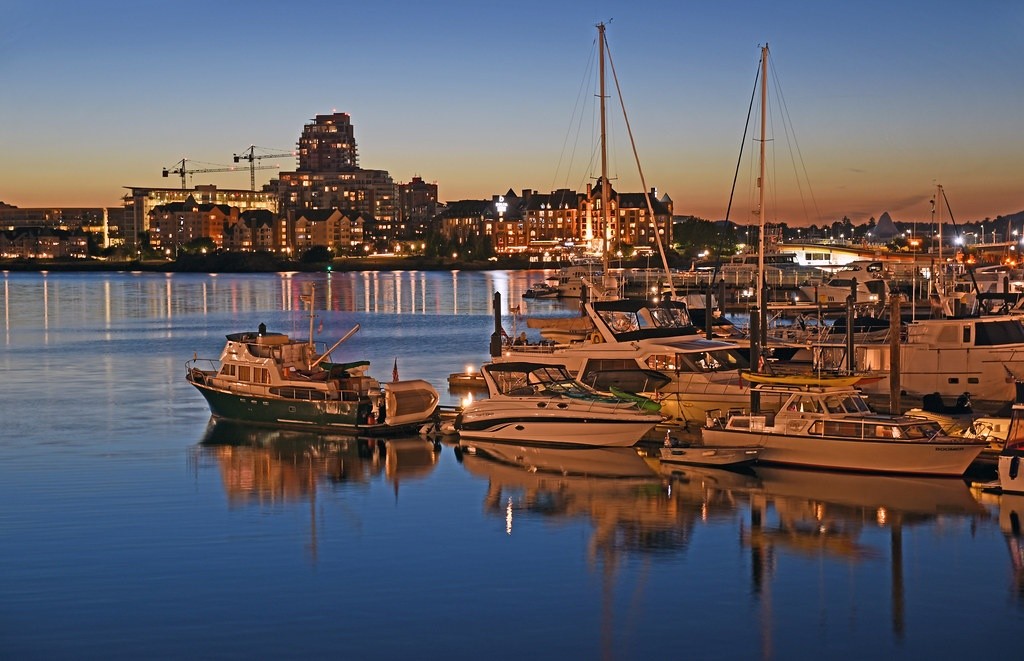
[
  {"left": 233, "top": 144, "right": 295, "bottom": 162},
  {"left": 162, "top": 157, "right": 280, "bottom": 189}
]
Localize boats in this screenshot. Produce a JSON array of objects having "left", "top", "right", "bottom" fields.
[
  {"left": 446, "top": 19, "right": 1023, "bottom": 494},
  {"left": 183, "top": 280, "right": 457, "bottom": 437}
]
